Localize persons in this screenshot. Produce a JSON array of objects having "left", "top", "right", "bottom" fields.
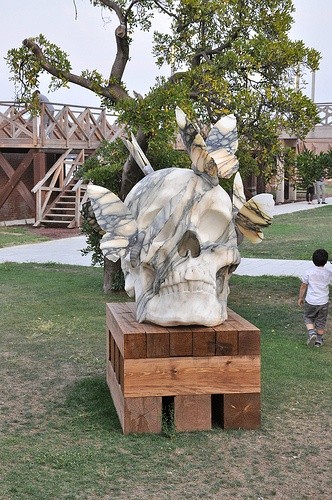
[
  {"left": 316, "top": 175, "right": 328, "bottom": 204},
  {"left": 298, "top": 249, "right": 332, "bottom": 347},
  {"left": 306, "top": 182, "right": 315, "bottom": 204},
  {"left": 35, "top": 90, "right": 54, "bottom": 139}
]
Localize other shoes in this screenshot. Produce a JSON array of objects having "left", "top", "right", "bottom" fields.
[
  {"left": 318, "top": 202, "right": 320, "bottom": 204},
  {"left": 307, "top": 333, "right": 317, "bottom": 348},
  {"left": 315, "top": 338, "right": 326, "bottom": 348},
  {"left": 322, "top": 202, "right": 328, "bottom": 204}
]
